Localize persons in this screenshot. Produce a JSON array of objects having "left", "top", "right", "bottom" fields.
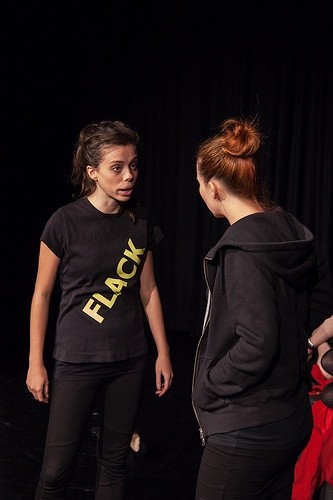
[
  {"left": 303, "top": 314, "right": 333, "bottom": 500},
  {"left": 26, "top": 121, "right": 174, "bottom": 500},
  {"left": 190, "top": 119, "right": 315, "bottom": 500}
]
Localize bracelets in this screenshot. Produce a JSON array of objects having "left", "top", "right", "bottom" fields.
[{"left": 307, "top": 338, "right": 316, "bottom": 352}]
